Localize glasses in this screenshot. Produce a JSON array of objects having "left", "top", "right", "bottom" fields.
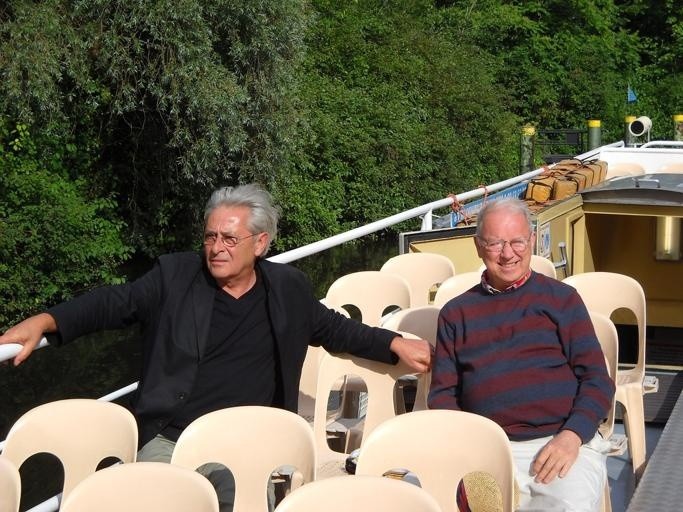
[
  {"left": 478, "top": 233, "right": 532, "bottom": 253},
  {"left": 202, "top": 231, "right": 261, "bottom": 247}
]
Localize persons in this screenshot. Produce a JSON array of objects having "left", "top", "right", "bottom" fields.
[
  {"left": 427, "top": 200, "right": 616, "bottom": 511},
  {"left": 0, "top": 183, "right": 433, "bottom": 509}
]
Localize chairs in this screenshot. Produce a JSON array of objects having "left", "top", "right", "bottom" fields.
[
  {"left": 326, "top": 270, "right": 411, "bottom": 454},
  {"left": 586, "top": 311, "right": 620, "bottom": 512},
  {"left": 312, "top": 329, "right": 432, "bottom": 473},
  {"left": 380, "top": 307, "right": 440, "bottom": 412},
  {"left": 434, "top": 271, "right": 483, "bottom": 313},
  {"left": 60, "top": 462, "right": 219, "bottom": 512},
  {"left": 170, "top": 405, "right": 317, "bottom": 512},
  {"left": 561, "top": 272, "right": 650, "bottom": 492},
  {"left": 298, "top": 306, "right": 352, "bottom": 433},
  {"left": 355, "top": 409, "right": 514, "bottom": 512},
  {"left": 0, "top": 457, "right": 22, "bottom": 512},
  {"left": 379, "top": 253, "right": 455, "bottom": 307},
  {"left": 473, "top": 255, "right": 557, "bottom": 294},
  {"left": 274, "top": 474, "right": 442, "bottom": 512},
  {"left": 1, "top": 398, "right": 138, "bottom": 512}
]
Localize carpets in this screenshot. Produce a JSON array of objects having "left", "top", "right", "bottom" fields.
[{"left": 603, "top": 366, "right": 683, "bottom": 428}]
